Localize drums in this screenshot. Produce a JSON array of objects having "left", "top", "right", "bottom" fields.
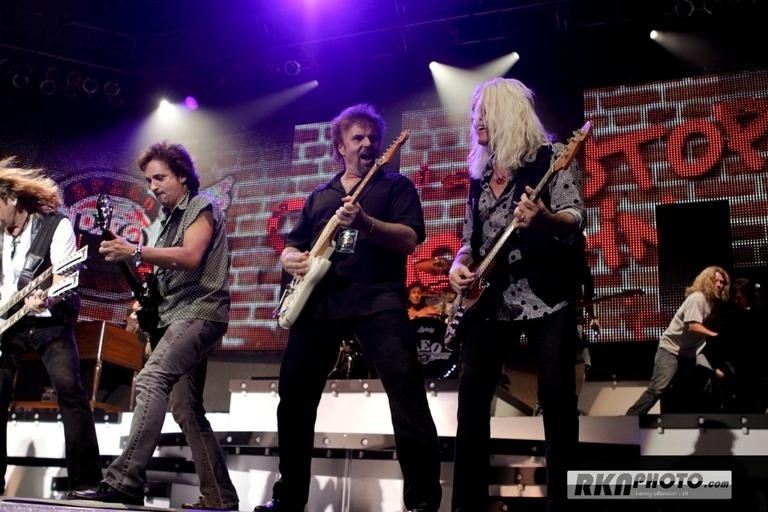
[{"left": 409, "top": 302, "right": 457, "bottom": 380}]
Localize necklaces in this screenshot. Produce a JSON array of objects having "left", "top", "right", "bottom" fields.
[
  {"left": 3, "top": 212, "right": 30, "bottom": 259},
  {"left": 492, "top": 171, "right": 510, "bottom": 186}
]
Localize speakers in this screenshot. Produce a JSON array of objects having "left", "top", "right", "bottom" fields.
[{"left": 656, "top": 200, "right": 737, "bottom": 289}]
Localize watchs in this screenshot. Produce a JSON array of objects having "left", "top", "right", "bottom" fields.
[{"left": 131, "top": 243, "right": 143, "bottom": 268}]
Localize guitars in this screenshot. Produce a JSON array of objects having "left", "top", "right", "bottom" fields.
[
  {"left": 97, "top": 189, "right": 166, "bottom": 353},
  {"left": 273, "top": 128, "right": 412, "bottom": 328},
  {"left": 0, "top": 243, "right": 88, "bottom": 321},
  {"left": 443, "top": 121, "right": 594, "bottom": 352},
  {"left": 0, "top": 269, "right": 79, "bottom": 334}
]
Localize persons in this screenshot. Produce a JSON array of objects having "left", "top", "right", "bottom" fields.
[
  {"left": 450, "top": 77, "right": 596, "bottom": 512},
  {"left": 0, "top": 154, "right": 105, "bottom": 502},
  {"left": 626, "top": 265, "right": 739, "bottom": 417},
  {"left": 408, "top": 281, "right": 426, "bottom": 320},
  {"left": 67, "top": 141, "right": 240, "bottom": 511},
  {"left": 254, "top": 102, "right": 443, "bottom": 511},
  {"left": 729, "top": 279, "right": 762, "bottom": 310},
  {"left": 442, "top": 287, "right": 458, "bottom": 303}
]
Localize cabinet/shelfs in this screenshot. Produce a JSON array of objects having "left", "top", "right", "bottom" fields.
[{"left": 8, "top": 319, "right": 145, "bottom": 411}]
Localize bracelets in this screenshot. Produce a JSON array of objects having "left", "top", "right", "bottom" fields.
[{"left": 368, "top": 215, "right": 376, "bottom": 237}]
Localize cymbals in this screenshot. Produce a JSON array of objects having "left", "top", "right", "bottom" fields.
[{"left": 414, "top": 257, "right": 451, "bottom": 275}]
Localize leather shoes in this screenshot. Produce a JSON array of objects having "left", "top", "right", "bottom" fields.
[
  {"left": 254, "top": 499, "right": 307, "bottom": 511},
  {"left": 66, "top": 485, "right": 145, "bottom": 505},
  {"left": 181, "top": 497, "right": 238, "bottom": 510}
]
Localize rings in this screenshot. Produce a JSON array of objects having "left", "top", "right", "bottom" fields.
[{"left": 520, "top": 214, "right": 525, "bottom": 222}]
